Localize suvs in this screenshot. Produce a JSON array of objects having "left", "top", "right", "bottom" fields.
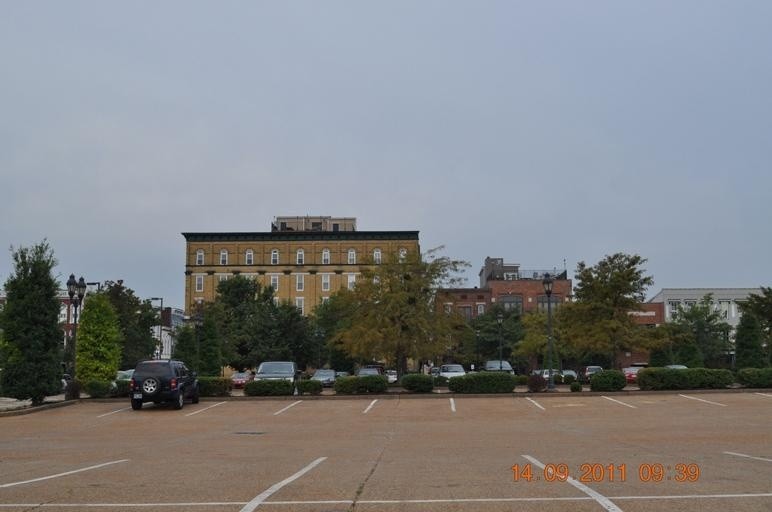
[{"left": 129, "top": 359, "right": 200, "bottom": 411}]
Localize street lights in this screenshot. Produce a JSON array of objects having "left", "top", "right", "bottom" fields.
[
  {"left": 151, "top": 298, "right": 164, "bottom": 358},
  {"left": 544, "top": 271, "right": 555, "bottom": 390},
  {"left": 67, "top": 272, "right": 85, "bottom": 380},
  {"left": 88, "top": 281, "right": 101, "bottom": 290},
  {"left": 476, "top": 327, "right": 480, "bottom": 372},
  {"left": 194, "top": 311, "right": 203, "bottom": 350},
  {"left": 496, "top": 310, "right": 503, "bottom": 371}
]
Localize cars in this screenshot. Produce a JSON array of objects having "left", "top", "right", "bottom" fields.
[
  {"left": 430, "top": 359, "right": 515, "bottom": 386},
  {"left": 664, "top": 364, "right": 688, "bottom": 367},
  {"left": 625, "top": 371, "right": 638, "bottom": 383},
  {"left": 111, "top": 368, "right": 135, "bottom": 394},
  {"left": 531, "top": 366, "right": 605, "bottom": 384},
  {"left": 226, "top": 360, "right": 397, "bottom": 389}
]
